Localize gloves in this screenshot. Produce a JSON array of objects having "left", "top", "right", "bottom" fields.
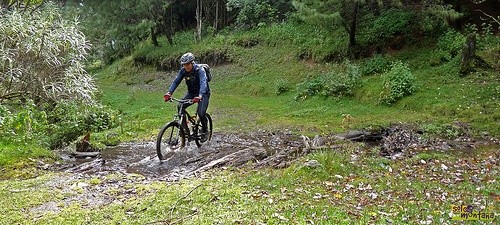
[
  {"left": 192, "top": 96, "right": 200, "bottom": 104},
  {"left": 164, "top": 93, "right": 171, "bottom": 102}
]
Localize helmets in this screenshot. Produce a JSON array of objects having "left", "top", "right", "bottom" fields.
[{"left": 181, "top": 53, "right": 195, "bottom": 64}]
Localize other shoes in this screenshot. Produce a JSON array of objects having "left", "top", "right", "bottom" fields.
[{"left": 200, "top": 130, "right": 209, "bottom": 143}]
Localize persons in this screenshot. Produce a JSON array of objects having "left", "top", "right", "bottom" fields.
[{"left": 164, "top": 53, "right": 210, "bottom": 145}]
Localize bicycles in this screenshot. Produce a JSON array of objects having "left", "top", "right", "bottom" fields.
[{"left": 156, "top": 95, "right": 213, "bottom": 161}]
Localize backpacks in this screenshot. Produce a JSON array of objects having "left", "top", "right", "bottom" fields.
[{"left": 198, "top": 64, "right": 212, "bottom": 82}]
